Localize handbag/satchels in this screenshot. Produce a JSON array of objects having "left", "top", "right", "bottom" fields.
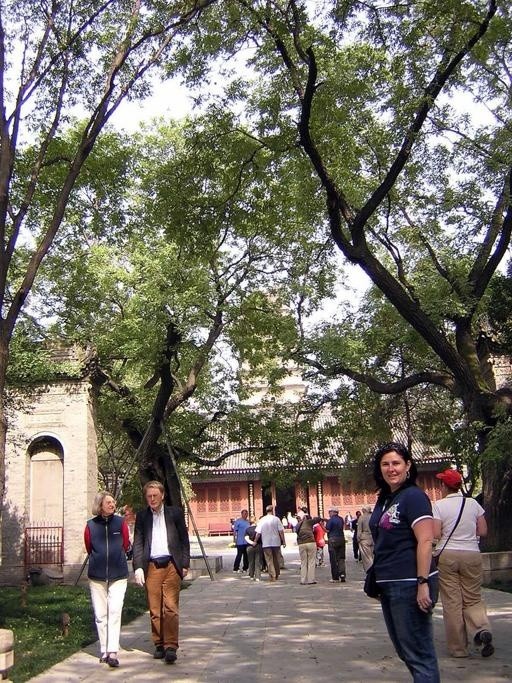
[
  {"left": 432, "top": 553, "right": 439, "bottom": 567},
  {"left": 153, "top": 556, "right": 170, "bottom": 569},
  {"left": 362, "top": 562, "right": 381, "bottom": 599}
]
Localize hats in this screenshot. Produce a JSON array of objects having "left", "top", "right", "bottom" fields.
[
  {"left": 434, "top": 468, "right": 462, "bottom": 489},
  {"left": 361, "top": 504, "right": 372, "bottom": 512},
  {"left": 326, "top": 506, "right": 340, "bottom": 512}
]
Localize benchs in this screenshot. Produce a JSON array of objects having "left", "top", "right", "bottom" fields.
[{"left": 208, "top": 524, "right": 233, "bottom": 536}]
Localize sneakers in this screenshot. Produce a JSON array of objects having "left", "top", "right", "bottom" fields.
[
  {"left": 340, "top": 574, "right": 346, "bottom": 582},
  {"left": 478, "top": 629, "right": 495, "bottom": 658},
  {"left": 99, "top": 651, "right": 109, "bottom": 663},
  {"left": 165, "top": 646, "right": 178, "bottom": 662},
  {"left": 233, "top": 569, "right": 281, "bottom": 582},
  {"left": 154, "top": 645, "right": 166, "bottom": 659},
  {"left": 108, "top": 654, "right": 121, "bottom": 667},
  {"left": 328, "top": 577, "right": 339, "bottom": 583}
]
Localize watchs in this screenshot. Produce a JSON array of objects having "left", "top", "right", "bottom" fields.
[{"left": 416, "top": 576, "right": 428, "bottom": 584}]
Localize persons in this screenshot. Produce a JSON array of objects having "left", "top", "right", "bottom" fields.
[
  {"left": 84, "top": 490, "right": 129, "bottom": 667},
  {"left": 132, "top": 480, "right": 190, "bottom": 664},
  {"left": 369, "top": 441, "right": 441, "bottom": 683},
  {"left": 232, "top": 505, "right": 375, "bottom": 584},
  {"left": 432, "top": 469, "right": 495, "bottom": 659}
]
